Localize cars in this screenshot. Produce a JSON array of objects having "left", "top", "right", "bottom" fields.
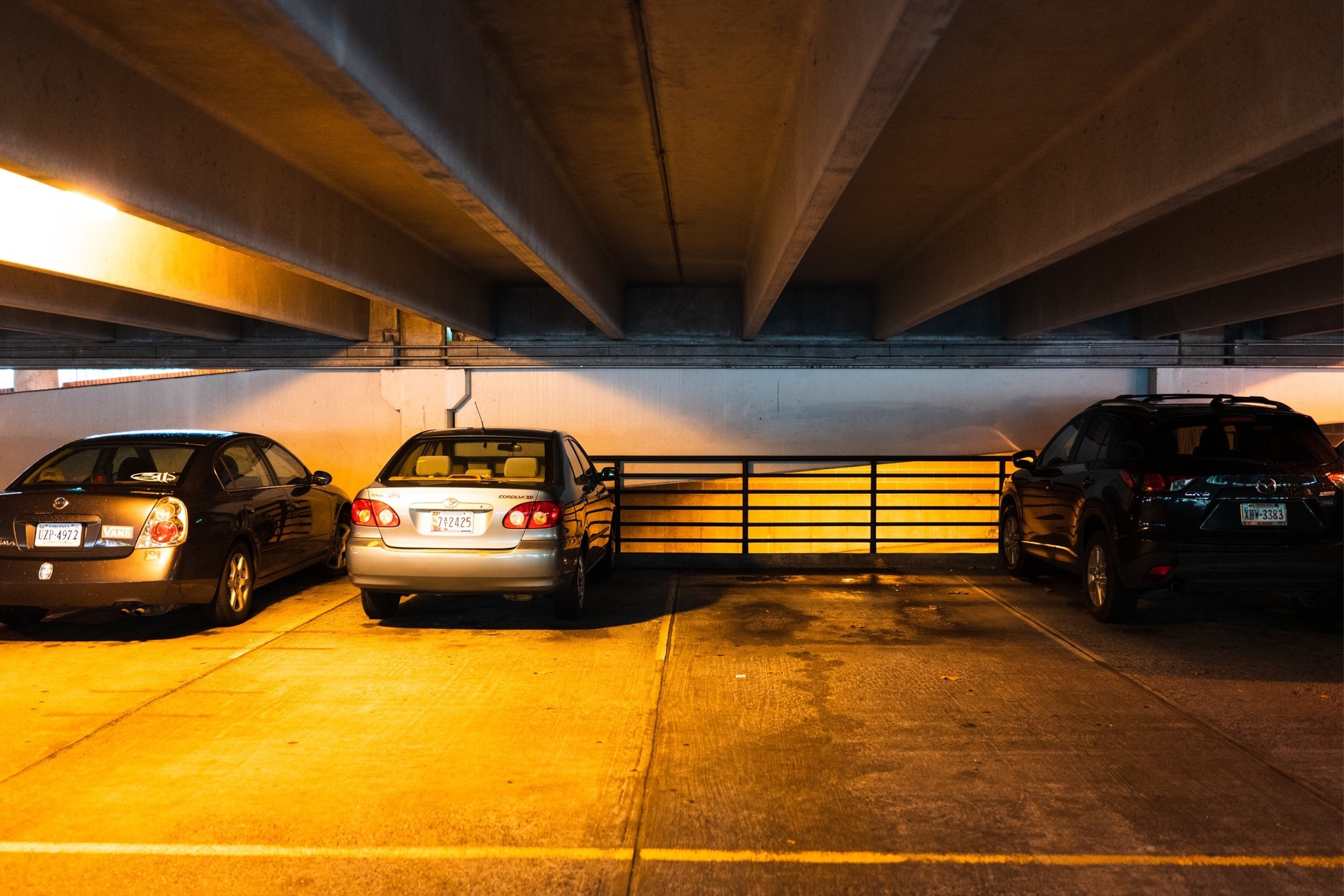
[
  {"left": 998, "top": 388, "right": 1344, "bottom": 623},
  {"left": 0, "top": 430, "right": 355, "bottom": 628},
  {"left": 346, "top": 425, "right": 621, "bottom": 622}
]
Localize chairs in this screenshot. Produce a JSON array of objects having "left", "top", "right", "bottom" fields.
[
  {"left": 1193, "top": 426, "right": 1234, "bottom": 461},
  {"left": 218, "top": 456, "right": 263, "bottom": 492},
  {"left": 417, "top": 455, "right": 452, "bottom": 478},
  {"left": 118, "top": 457, "right": 152, "bottom": 483},
  {"left": 504, "top": 458, "right": 539, "bottom": 478}
]
